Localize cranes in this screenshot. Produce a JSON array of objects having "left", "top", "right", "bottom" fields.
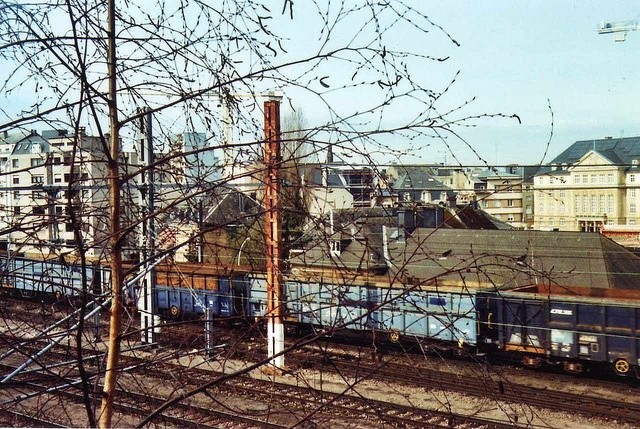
[{"left": 98, "top": 74, "right": 283, "bottom": 175}]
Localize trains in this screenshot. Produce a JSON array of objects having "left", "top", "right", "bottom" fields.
[{"left": 0, "top": 250, "right": 640, "bottom": 379}]
